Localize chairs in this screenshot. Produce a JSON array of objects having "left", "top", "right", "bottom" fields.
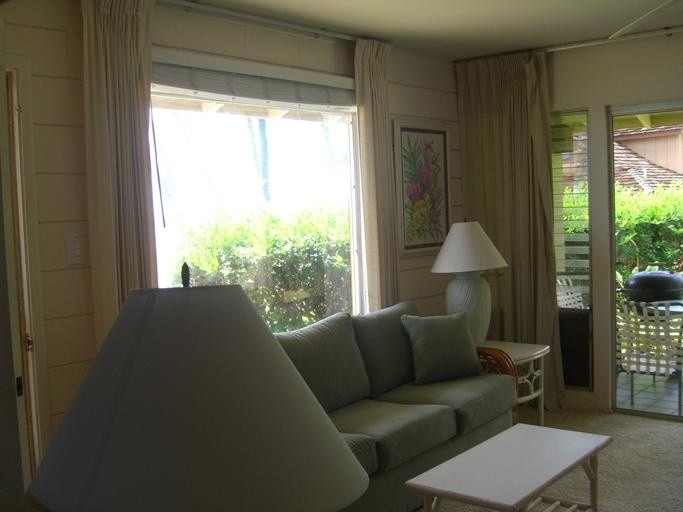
[
  {"left": 611, "top": 293, "right": 682, "bottom": 416},
  {"left": 551, "top": 274, "right": 588, "bottom": 309}
]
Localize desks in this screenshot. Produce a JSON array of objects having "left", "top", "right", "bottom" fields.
[{"left": 481, "top": 334, "right": 553, "bottom": 425}]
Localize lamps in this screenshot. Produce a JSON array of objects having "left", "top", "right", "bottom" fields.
[
  {"left": 430, "top": 220, "right": 509, "bottom": 347},
  {"left": 21, "top": 283, "right": 373, "bottom": 511}
]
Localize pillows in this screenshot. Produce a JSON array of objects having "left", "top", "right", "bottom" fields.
[{"left": 399, "top": 311, "right": 479, "bottom": 386}]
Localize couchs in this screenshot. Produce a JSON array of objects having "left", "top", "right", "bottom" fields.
[{"left": 247, "top": 299, "right": 518, "bottom": 509}]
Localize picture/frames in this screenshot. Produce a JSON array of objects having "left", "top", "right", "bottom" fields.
[{"left": 391, "top": 115, "right": 455, "bottom": 260}]
